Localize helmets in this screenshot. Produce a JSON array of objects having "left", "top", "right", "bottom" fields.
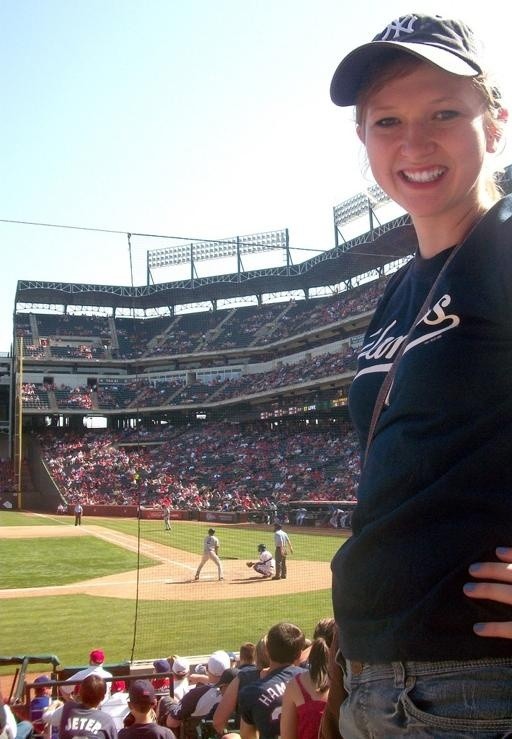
[
  {"left": 258, "top": 544, "right": 266, "bottom": 551},
  {"left": 208, "top": 528, "right": 215, "bottom": 534}
]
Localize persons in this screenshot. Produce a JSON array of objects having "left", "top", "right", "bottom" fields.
[
  {"left": 213, "top": 634, "right": 271, "bottom": 739},
  {"left": 282, "top": 616, "right": 337, "bottom": 739},
  {"left": 176, "top": 298, "right": 301, "bottom": 351},
  {"left": 74, "top": 501, "right": 83, "bottom": 526},
  {"left": 28, "top": 677, "right": 59, "bottom": 739},
  {"left": 100, "top": 680, "right": 131, "bottom": 732},
  {"left": 16, "top": 314, "right": 178, "bottom": 359},
  {"left": 166, "top": 651, "right": 230, "bottom": 728},
  {"left": 0, "top": 458, "right": 17, "bottom": 509},
  {"left": 62, "top": 503, "right": 68, "bottom": 515},
  {"left": 129, "top": 379, "right": 233, "bottom": 408},
  {"left": 243, "top": 503, "right": 354, "bottom": 528},
  {"left": 56, "top": 502, "right": 64, "bottom": 516},
  {"left": 229, "top": 342, "right": 361, "bottom": 398},
  {"left": 118, "top": 680, "right": 176, "bottom": 739},
  {"left": 251, "top": 544, "right": 275, "bottom": 578},
  {"left": 195, "top": 528, "right": 224, "bottom": 581},
  {"left": 19, "top": 379, "right": 143, "bottom": 410},
  {"left": 61, "top": 650, "right": 114, "bottom": 706},
  {"left": 272, "top": 523, "right": 294, "bottom": 580},
  {"left": 161, "top": 504, "right": 173, "bottom": 530},
  {"left": 170, "top": 657, "right": 189, "bottom": 705},
  {"left": 235, "top": 641, "right": 256, "bottom": 670},
  {"left": 215, "top": 668, "right": 242, "bottom": 732},
  {"left": 227, "top": 652, "right": 238, "bottom": 668},
  {"left": 58, "top": 674, "right": 118, "bottom": 739},
  {"left": 147, "top": 660, "right": 170, "bottom": 696},
  {"left": 236, "top": 622, "right": 307, "bottom": 739},
  {"left": 38, "top": 419, "right": 361, "bottom": 510},
  {"left": 297, "top": 273, "right": 394, "bottom": 333},
  {"left": 0, "top": 689, "right": 17, "bottom": 739},
  {"left": 330, "top": 14, "right": 512, "bottom": 739}
]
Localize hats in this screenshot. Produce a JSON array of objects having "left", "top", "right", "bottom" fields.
[
  {"left": 90, "top": 650, "right": 104, "bottom": 666},
  {"left": 153, "top": 658, "right": 170, "bottom": 672},
  {"left": 129, "top": 678, "right": 156, "bottom": 703},
  {"left": 111, "top": 679, "right": 125, "bottom": 692},
  {"left": 215, "top": 668, "right": 238, "bottom": 687},
  {"left": 33, "top": 675, "right": 54, "bottom": 687},
  {"left": 173, "top": 658, "right": 189, "bottom": 675},
  {"left": 331, "top": 16, "right": 487, "bottom": 107},
  {"left": 207, "top": 650, "right": 231, "bottom": 675}
]
